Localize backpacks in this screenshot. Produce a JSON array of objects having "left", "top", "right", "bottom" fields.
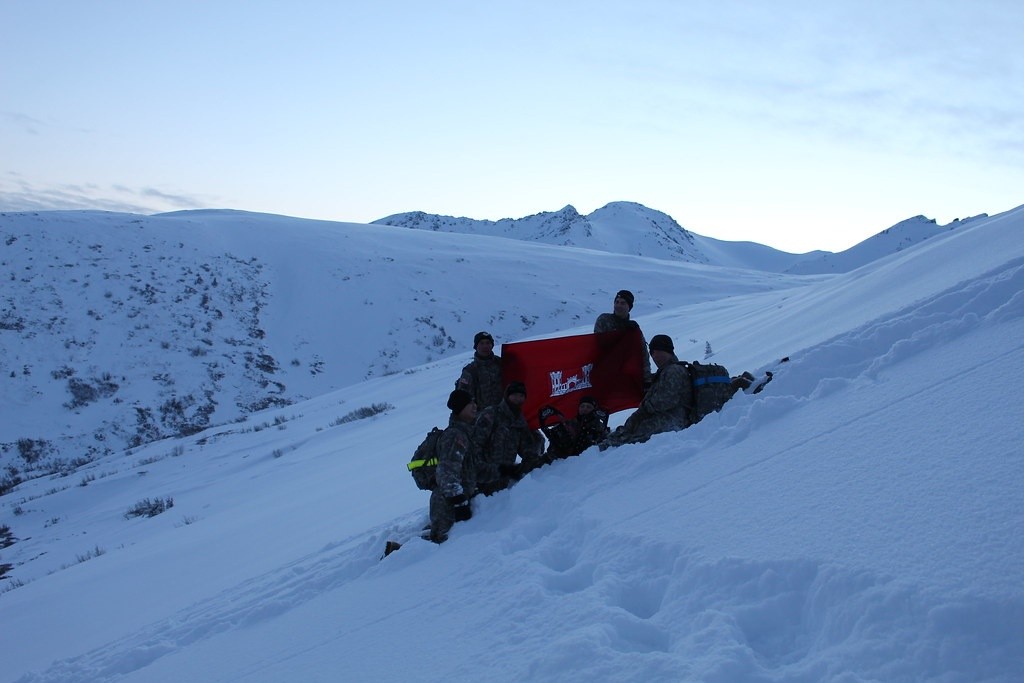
[
  {"left": 407, "top": 427, "right": 475, "bottom": 490},
  {"left": 656, "top": 362, "right": 730, "bottom": 424}
]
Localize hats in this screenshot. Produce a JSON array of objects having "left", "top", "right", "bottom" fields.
[
  {"left": 649, "top": 335, "right": 674, "bottom": 352},
  {"left": 505, "top": 382, "right": 527, "bottom": 400},
  {"left": 474, "top": 332, "right": 494, "bottom": 350},
  {"left": 614, "top": 290, "right": 634, "bottom": 311},
  {"left": 579, "top": 396, "right": 596, "bottom": 407},
  {"left": 447, "top": 390, "right": 473, "bottom": 413}
]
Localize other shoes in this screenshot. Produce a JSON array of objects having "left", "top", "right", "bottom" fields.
[{"left": 380, "top": 541, "right": 401, "bottom": 561}]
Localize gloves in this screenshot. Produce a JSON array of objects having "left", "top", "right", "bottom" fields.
[
  {"left": 454, "top": 500, "right": 470, "bottom": 521},
  {"left": 498, "top": 462, "right": 521, "bottom": 476},
  {"left": 513, "top": 463, "right": 532, "bottom": 479},
  {"left": 643, "top": 382, "right": 651, "bottom": 396},
  {"left": 624, "top": 320, "right": 639, "bottom": 332}
]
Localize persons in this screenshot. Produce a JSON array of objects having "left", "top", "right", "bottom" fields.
[
  {"left": 380, "top": 390, "right": 481, "bottom": 558},
  {"left": 472, "top": 380, "right": 543, "bottom": 496},
  {"left": 594, "top": 290, "right": 652, "bottom": 401},
  {"left": 457, "top": 332, "right": 502, "bottom": 414},
  {"left": 607, "top": 334, "right": 689, "bottom": 447},
  {"left": 538, "top": 395, "right": 608, "bottom": 468}
]
